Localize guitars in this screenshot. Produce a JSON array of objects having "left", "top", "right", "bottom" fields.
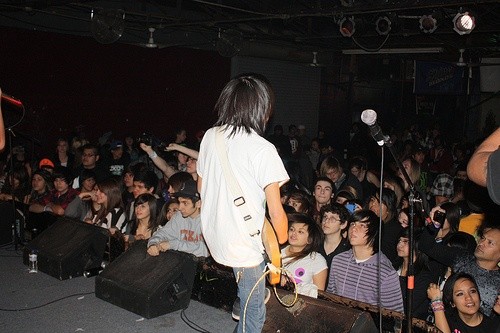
[{"left": 259, "top": 214, "right": 284, "bottom": 286}]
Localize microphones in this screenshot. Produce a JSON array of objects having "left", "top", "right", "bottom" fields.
[{"left": 361, "top": 109, "right": 386, "bottom": 146}]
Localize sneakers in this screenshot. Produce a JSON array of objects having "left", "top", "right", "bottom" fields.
[{"left": 231, "top": 288, "right": 270, "bottom": 321}]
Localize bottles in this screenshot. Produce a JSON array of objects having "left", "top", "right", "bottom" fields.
[{"left": 28, "top": 250, "right": 37, "bottom": 274}]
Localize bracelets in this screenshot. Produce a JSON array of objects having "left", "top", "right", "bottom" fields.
[
  {"left": 429, "top": 297, "right": 446, "bottom": 312},
  {"left": 149, "top": 244, "right": 157, "bottom": 248},
  {"left": 149, "top": 151, "right": 157, "bottom": 160},
  {"left": 44, "top": 205, "right": 48, "bottom": 211}
]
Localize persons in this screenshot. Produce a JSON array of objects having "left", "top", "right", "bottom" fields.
[
  {"left": 466, "top": 126, "right": 500, "bottom": 188},
  {"left": 426, "top": 272, "right": 500, "bottom": 333},
  {"left": 386, "top": 225, "right": 442, "bottom": 326},
  {"left": 438, "top": 227, "right": 500, "bottom": 317},
  {"left": 0, "top": 124, "right": 500, "bottom": 291},
  {"left": 196, "top": 76, "right": 291, "bottom": 333},
  {"left": 0, "top": 87, "right": 5, "bottom": 149},
  {"left": 324, "top": 208, "right": 404, "bottom": 333}
]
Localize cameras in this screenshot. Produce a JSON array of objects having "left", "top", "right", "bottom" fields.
[
  {"left": 344, "top": 204, "right": 357, "bottom": 214},
  {"left": 433, "top": 211, "right": 444, "bottom": 223}
]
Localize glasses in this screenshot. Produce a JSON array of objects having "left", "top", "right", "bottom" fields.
[
  {"left": 82, "top": 153, "right": 94, "bottom": 157},
  {"left": 398, "top": 239, "right": 409, "bottom": 245},
  {"left": 322, "top": 215, "right": 341, "bottom": 223}
]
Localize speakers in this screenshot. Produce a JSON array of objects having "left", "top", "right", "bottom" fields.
[
  {"left": 22, "top": 218, "right": 110, "bottom": 281},
  {"left": 264, "top": 287, "right": 379, "bottom": 333},
  {"left": 0, "top": 203, "right": 16, "bottom": 245},
  {"left": 97, "top": 240, "right": 197, "bottom": 319}
]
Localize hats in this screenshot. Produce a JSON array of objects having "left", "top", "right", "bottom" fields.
[
  {"left": 39, "top": 159, "right": 54, "bottom": 169},
  {"left": 170, "top": 180, "right": 197, "bottom": 196},
  {"left": 432, "top": 173, "right": 454, "bottom": 197},
  {"left": 110, "top": 140, "right": 123, "bottom": 150}
]
